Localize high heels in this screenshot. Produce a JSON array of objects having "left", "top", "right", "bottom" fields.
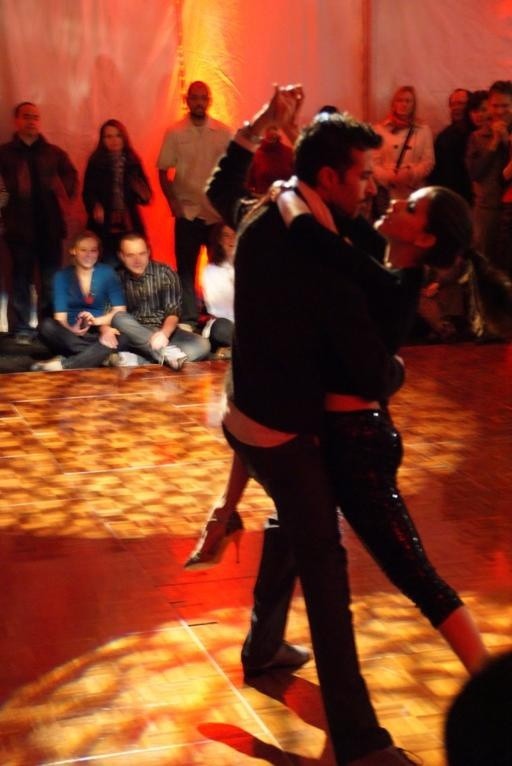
[{"left": 184, "top": 508, "right": 244, "bottom": 571}]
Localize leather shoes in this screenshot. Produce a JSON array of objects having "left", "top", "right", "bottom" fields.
[
  {"left": 347, "top": 747, "right": 424, "bottom": 766},
  {"left": 243, "top": 640, "right": 313, "bottom": 675}
]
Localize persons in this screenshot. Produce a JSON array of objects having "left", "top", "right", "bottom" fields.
[
  {"left": 98, "top": 233, "right": 211, "bottom": 373},
  {"left": 243, "top": 103, "right": 340, "bottom": 194},
  {"left": 181, "top": 79, "right": 497, "bottom": 682},
  {"left": 82, "top": 117, "right": 151, "bottom": 265},
  {"left": 201, "top": 83, "right": 423, "bottom": 765},
  {"left": 0, "top": 103, "right": 78, "bottom": 344},
  {"left": 31, "top": 231, "right": 129, "bottom": 372},
  {"left": 370, "top": 77, "right": 512, "bottom": 345},
  {"left": 198, "top": 222, "right": 236, "bottom": 358},
  {"left": 158, "top": 80, "right": 236, "bottom": 314}
]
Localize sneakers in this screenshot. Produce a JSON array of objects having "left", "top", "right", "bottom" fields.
[
  {"left": 109, "top": 351, "right": 151, "bottom": 366},
  {"left": 30, "top": 354, "right": 65, "bottom": 371},
  {"left": 157, "top": 343, "right": 188, "bottom": 370}
]
